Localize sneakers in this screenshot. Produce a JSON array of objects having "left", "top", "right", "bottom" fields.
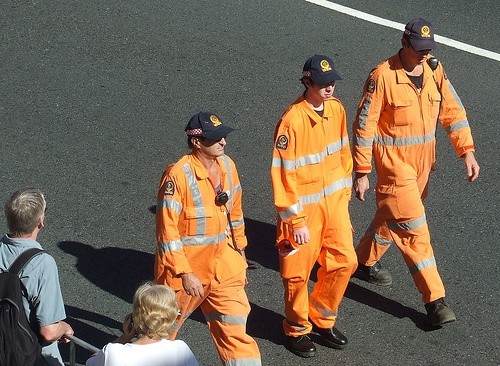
[
  {"left": 424, "top": 298, "right": 456, "bottom": 327},
  {"left": 356, "top": 261, "right": 392, "bottom": 286}
]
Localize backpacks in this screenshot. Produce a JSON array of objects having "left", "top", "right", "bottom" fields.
[{"left": 0, "top": 248, "right": 49, "bottom": 366}]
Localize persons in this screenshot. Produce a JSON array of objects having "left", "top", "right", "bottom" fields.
[
  {"left": 271, "top": 54, "right": 359, "bottom": 358},
  {"left": 155, "top": 112, "right": 261, "bottom": 366},
  {"left": 353, "top": 17, "right": 480, "bottom": 326},
  {"left": 0, "top": 188, "right": 74, "bottom": 366},
  {"left": 86, "top": 282, "right": 199, "bottom": 366}
]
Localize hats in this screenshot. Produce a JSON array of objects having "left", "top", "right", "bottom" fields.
[
  {"left": 186, "top": 111, "right": 235, "bottom": 139},
  {"left": 405, "top": 18, "right": 436, "bottom": 51},
  {"left": 302, "top": 54, "right": 343, "bottom": 85}
]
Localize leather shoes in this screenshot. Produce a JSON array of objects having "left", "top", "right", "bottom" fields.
[
  {"left": 290, "top": 334, "right": 316, "bottom": 357},
  {"left": 311, "top": 325, "right": 348, "bottom": 348}
]
